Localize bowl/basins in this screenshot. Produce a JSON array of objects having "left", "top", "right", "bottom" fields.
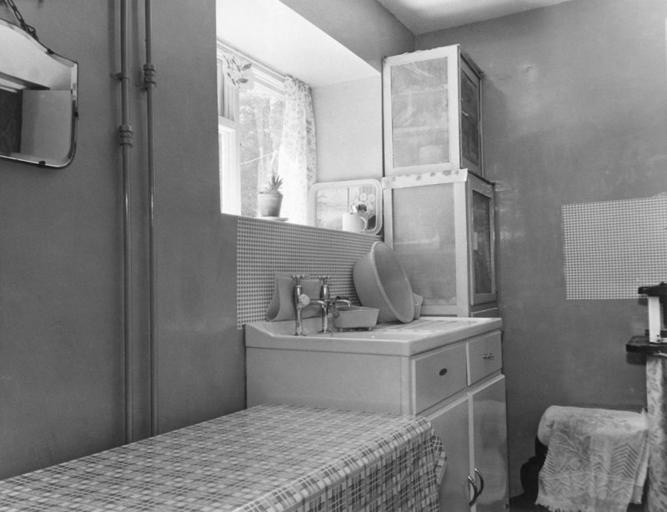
[{"left": 353, "top": 240, "right": 415, "bottom": 324}]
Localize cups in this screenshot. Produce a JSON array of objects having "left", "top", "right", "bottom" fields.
[{"left": 343, "top": 215, "right": 367, "bottom": 233}]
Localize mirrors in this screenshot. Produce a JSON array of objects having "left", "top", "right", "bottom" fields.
[{"left": 0, "top": 18, "right": 79, "bottom": 170}]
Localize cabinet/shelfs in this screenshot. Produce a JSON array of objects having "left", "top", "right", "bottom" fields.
[
  {"left": 380, "top": 42, "right": 499, "bottom": 316},
  {"left": 245, "top": 329, "right": 511, "bottom": 511}
]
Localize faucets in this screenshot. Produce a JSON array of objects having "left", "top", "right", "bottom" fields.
[
  {"left": 291, "top": 274, "right": 328, "bottom": 336},
  {"left": 317, "top": 274, "right": 352, "bottom": 333}
]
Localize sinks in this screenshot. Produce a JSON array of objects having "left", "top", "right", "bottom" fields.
[{"left": 314, "top": 328, "right": 418, "bottom": 341}]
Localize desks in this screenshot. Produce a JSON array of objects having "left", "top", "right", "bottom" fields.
[{"left": 0, "top": 401, "right": 432, "bottom": 511}]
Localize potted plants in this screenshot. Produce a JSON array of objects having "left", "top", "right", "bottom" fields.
[{"left": 257, "top": 174, "right": 283, "bottom": 216}]
